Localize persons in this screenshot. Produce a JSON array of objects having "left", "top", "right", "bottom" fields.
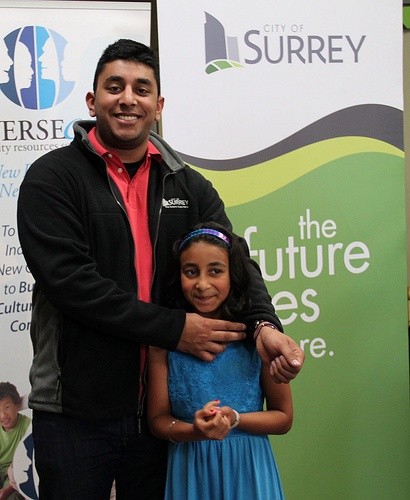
[
  {"left": 148, "top": 222, "right": 296, "bottom": 499},
  {"left": 0, "top": 382, "right": 32, "bottom": 499},
  {"left": 17, "top": 38, "right": 307, "bottom": 499}
]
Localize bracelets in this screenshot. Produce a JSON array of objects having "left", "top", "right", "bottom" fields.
[
  {"left": 253, "top": 323, "right": 279, "bottom": 341},
  {"left": 167, "top": 417, "right": 184, "bottom": 446},
  {"left": 227, "top": 408, "right": 241, "bottom": 431}
]
